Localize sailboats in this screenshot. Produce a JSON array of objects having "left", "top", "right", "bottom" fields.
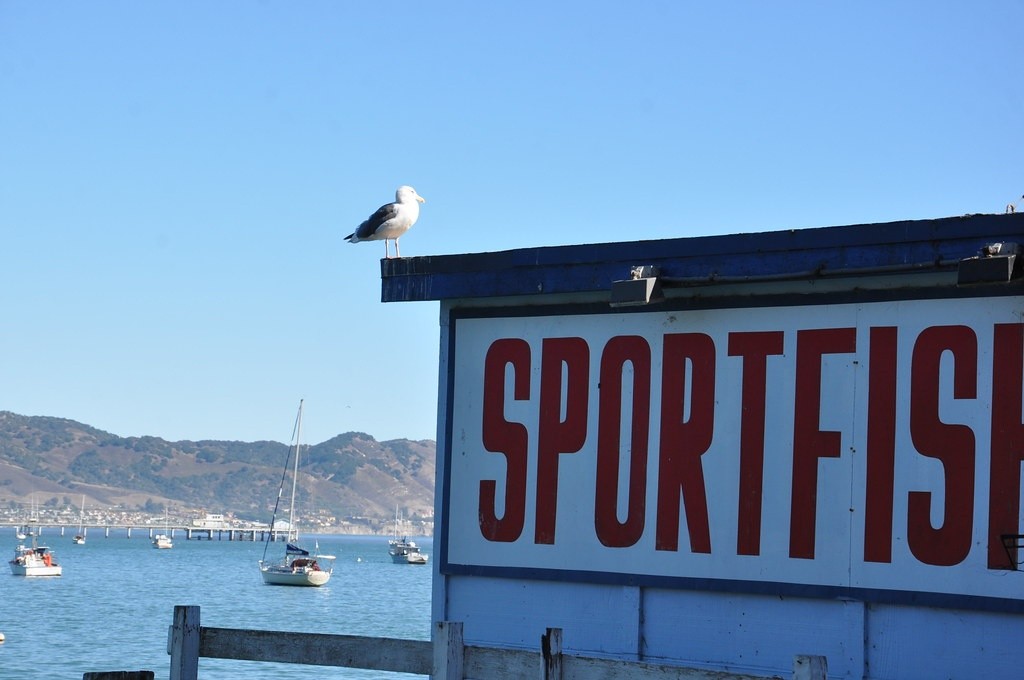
[
  {"left": 12, "top": 493, "right": 41, "bottom": 540},
  {"left": 71, "top": 494, "right": 88, "bottom": 545},
  {"left": 257, "top": 395, "right": 336, "bottom": 588}
]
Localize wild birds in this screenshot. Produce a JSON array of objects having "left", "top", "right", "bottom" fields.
[{"left": 342, "top": 184, "right": 425, "bottom": 260}]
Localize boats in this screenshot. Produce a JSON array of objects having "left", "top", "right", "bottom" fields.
[
  {"left": 151, "top": 533, "right": 173, "bottom": 550},
  {"left": 387, "top": 504, "right": 429, "bottom": 564},
  {"left": 7, "top": 537, "right": 63, "bottom": 577}
]
